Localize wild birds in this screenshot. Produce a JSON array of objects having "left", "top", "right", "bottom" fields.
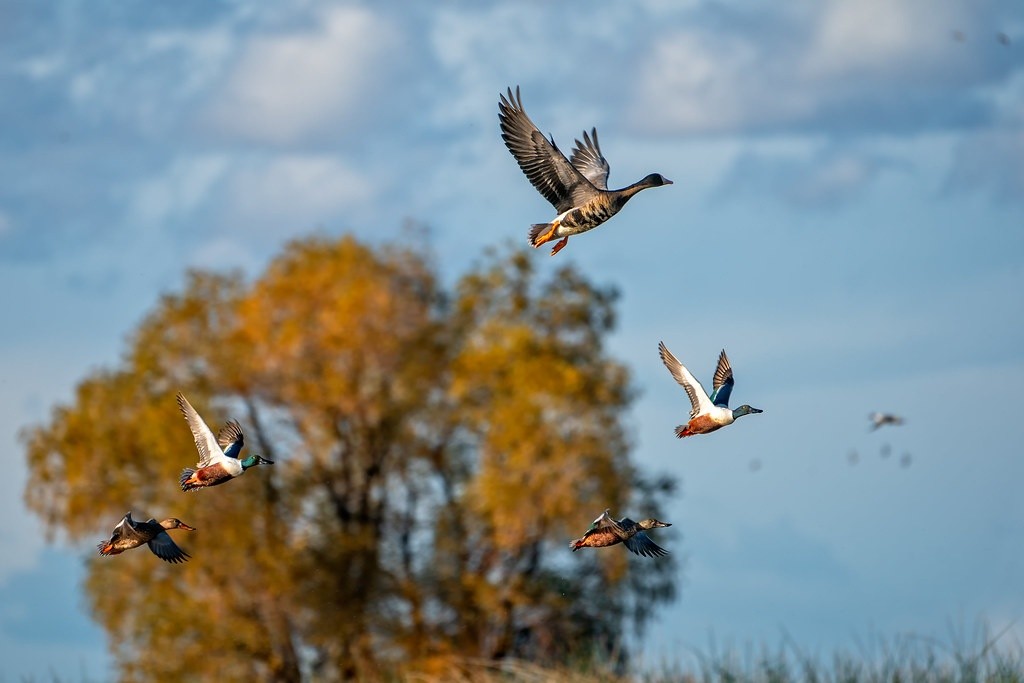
[
  {"left": 568, "top": 507, "right": 673, "bottom": 560},
  {"left": 498, "top": 87, "right": 674, "bottom": 257},
  {"left": 96, "top": 511, "right": 197, "bottom": 564},
  {"left": 864, "top": 409, "right": 909, "bottom": 434},
  {"left": 175, "top": 390, "right": 275, "bottom": 492},
  {"left": 657, "top": 340, "right": 764, "bottom": 438}
]
